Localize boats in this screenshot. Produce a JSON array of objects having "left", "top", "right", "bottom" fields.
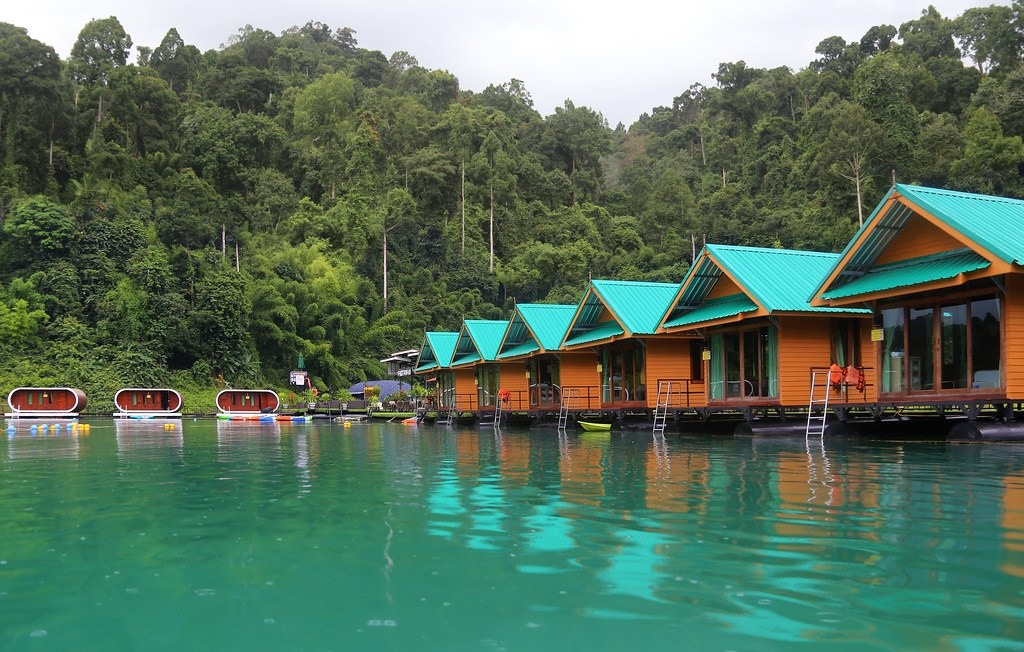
[
  {"left": 577, "top": 420, "right": 611, "bottom": 432},
  {"left": 400, "top": 416, "right": 417, "bottom": 423}
]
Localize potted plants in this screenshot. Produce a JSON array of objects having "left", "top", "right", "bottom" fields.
[{"left": 364, "top": 386, "right": 381, "bottom": 397}]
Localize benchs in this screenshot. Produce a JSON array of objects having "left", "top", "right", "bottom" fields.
[{"left": 346, "top": 401, "right": 371, "bottom": 409}]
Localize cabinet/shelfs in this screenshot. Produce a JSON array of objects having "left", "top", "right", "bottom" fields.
[
  {"left": 536, "top": 383, "right": 553, "bottom": 401},
  {"left": 415, "top": 396, "right": 438, "bottom": 410},
  {"left": 890, "top": 357, "right": 921, "bottom": 392},
  {"left": 609, "top": 377, "right": 622, "bottom": 401},
  {"left": 314, "top": 401, "right": 343, "bottom": 416},
  {"left": 728, "top": 371, "right": 740, "bottom": 396},
  {"left": 282, "top": 403, "right": 308, "bottom": 415}
]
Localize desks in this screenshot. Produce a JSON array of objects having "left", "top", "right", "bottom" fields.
[{"left": 409, "top": 401, "right": 417, "bottom": 411}]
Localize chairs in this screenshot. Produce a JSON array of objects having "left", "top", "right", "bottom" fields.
[{"left": 383, "top": 401, "right": 410, "bottom": 412}]
